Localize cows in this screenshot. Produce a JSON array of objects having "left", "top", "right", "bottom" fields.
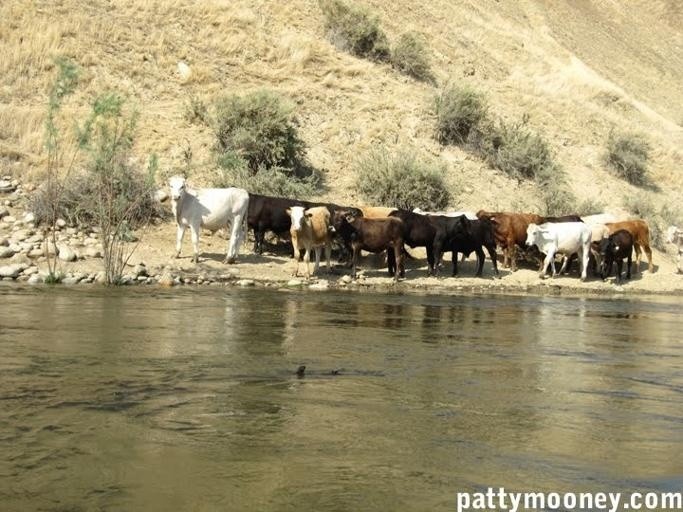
[
  {"left": 248, "top": 192, "right": 655, "bottom": 283},
  {"left": 664, "top": 225, "right": 683, "bottom": 274},
  {"left": 165, "top": 174, "right": 251, "bottom": 265}
]
